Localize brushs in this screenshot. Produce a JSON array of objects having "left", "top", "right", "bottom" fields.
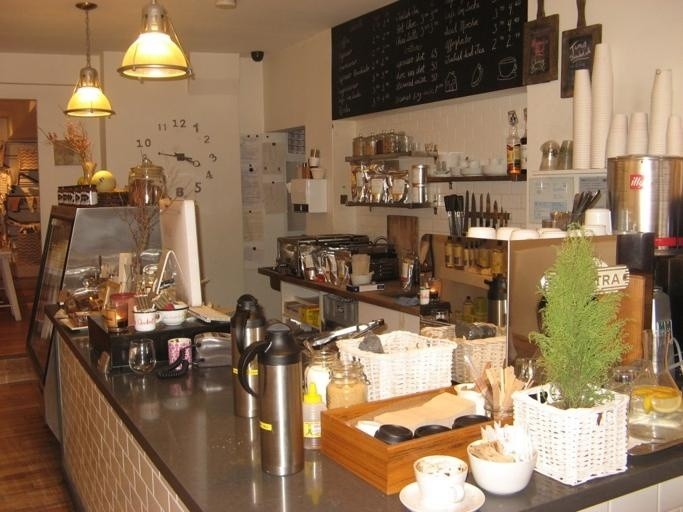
[{"left": 440, "top": 193, "right": 469, "bottom": 241}]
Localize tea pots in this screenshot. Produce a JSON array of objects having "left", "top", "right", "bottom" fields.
[{"left": 484, "top": 273, "right": 507, "bottom": 327}]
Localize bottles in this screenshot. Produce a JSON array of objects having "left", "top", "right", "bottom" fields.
[
  {"left": 304, "top": 350, "right": 369, "bottom": 451},
  {"left": 444, "top": 236, "right": 505, "bottom": 276},
  {"left": 353, "top": 127, "right": 412, "bottom": 156},
  {"left": 302, "top": 163, "right": 307, "bottom": 179},
  {"left": 231, "top": 294, "right": 306, "bottom": 478},
  {"left": 462, "top": 295, "right": 487, "bottom": 325},
  {"left": 627, "top": 329, "right": 683, "bottom": 445},
  {"left": 128, "top": 157, "right": 167, "bottom": 207},
  {"left": 505, "top": 108, "right": 528, "bottom": 174}
]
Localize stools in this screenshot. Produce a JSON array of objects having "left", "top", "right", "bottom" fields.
[{"left": 0, "top": 248, "right": 25, "bottom": 322}]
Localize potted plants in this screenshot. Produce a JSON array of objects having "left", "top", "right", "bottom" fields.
[
  {"left": 89, "top": 150, "right": 194, "bottom": 293},
  {"left": 36, "top": 104, "right": 97, "bottom": 186},
  {"left": 512, "top": 220, "right": 633, "bottom": 489}
]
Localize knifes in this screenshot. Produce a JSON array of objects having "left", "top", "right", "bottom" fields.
[{"left": 443, "top": 190, "right": 508, "bottom": 235}]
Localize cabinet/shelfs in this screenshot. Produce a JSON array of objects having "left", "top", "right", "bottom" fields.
[
  {"left": 279, "top": 280, "right": 321, "bottom": 338},
  {"left": 358, "top": 302, "right": 420, "bottom": 347},
  {"left": 344, "top": 145, "right": 438, "bottom": 214}
]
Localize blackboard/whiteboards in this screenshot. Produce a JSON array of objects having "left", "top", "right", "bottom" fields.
[{"left": 331, "top": 0, "right": 528, "bottom": 121}]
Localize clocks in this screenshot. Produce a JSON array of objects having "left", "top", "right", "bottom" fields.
[{"left": 134, "top": 113, "right": 219, "bottom": 200}]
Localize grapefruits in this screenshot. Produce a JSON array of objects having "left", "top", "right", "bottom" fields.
[{"left": 91, "top": 170, "right": 117, "bottom": 192}]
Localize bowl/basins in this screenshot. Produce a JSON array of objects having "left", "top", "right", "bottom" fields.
[
  {"left": 460, "top": 165, "right": 506, "bottom": 177},
  {"left": 157, "top": 304, "right": 189, "bottom": 326},
  {"left": 467, "top": 439, "right": 537, "bottom": 495}
]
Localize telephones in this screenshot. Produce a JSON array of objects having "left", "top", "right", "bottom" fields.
[{"left": 191, "top": 331, "right": 233, "bottom": 369}]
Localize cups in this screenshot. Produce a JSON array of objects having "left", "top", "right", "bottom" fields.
[
  {"left": 128, "top": 337, "right": 192, "bottom": 376},
  {"left": 105, "top": 301, "right": 163, "bottom": 332},
  {"left": 433, "top": 151, "right": 469, "bottom": 173},
  {"left": 539, "top": 140, "right": 572, "bottom": 170},
  {"left": 413, "top": 453, "right": 469, "bottom": 503},
  {"left": 515, "top": 356, "right": 646, "bottom": 390},
  {"left": 466, "top": 226, "right": 592, "bottom": 241},
  {"left": 484, "top": 401, "right": 512, "bottom": 426},
  {"left": 309, "top": 157, "right": 325, "bottom": 179},
  {"left": 571, "top": 42, "right": 683, "bottom": 169}
]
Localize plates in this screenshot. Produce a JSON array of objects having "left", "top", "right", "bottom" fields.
[{"left": 398, "top": 479, "right": 486, "bottom": 512}]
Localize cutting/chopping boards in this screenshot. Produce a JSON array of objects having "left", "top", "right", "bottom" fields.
[{"left": 387, "top": 215, "right": 419, "bottom": 279}]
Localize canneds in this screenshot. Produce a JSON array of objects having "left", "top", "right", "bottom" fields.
[
  {"left": 412, "top": 164, "right": 424, "bottom": 183},
  {"left": 412, "top": 185, "right": 425, "bottom": 204}
]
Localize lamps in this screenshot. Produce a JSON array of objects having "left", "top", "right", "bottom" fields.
[
  {"left": 116, "top": 0, "right": 194, "bottom": 82},
  {"left": 62, "top": 2, "right": 117, "bottom": 118}
]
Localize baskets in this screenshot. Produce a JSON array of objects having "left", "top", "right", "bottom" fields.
[
  {"left": 334, "top": 329, "right": 458, "bottom": 403},
  {"left": 512, "top": 378, "right": 631, "bottom": 487},
  {"left": 418, "top": 321, "right": 507, "bottom": 384}
]
